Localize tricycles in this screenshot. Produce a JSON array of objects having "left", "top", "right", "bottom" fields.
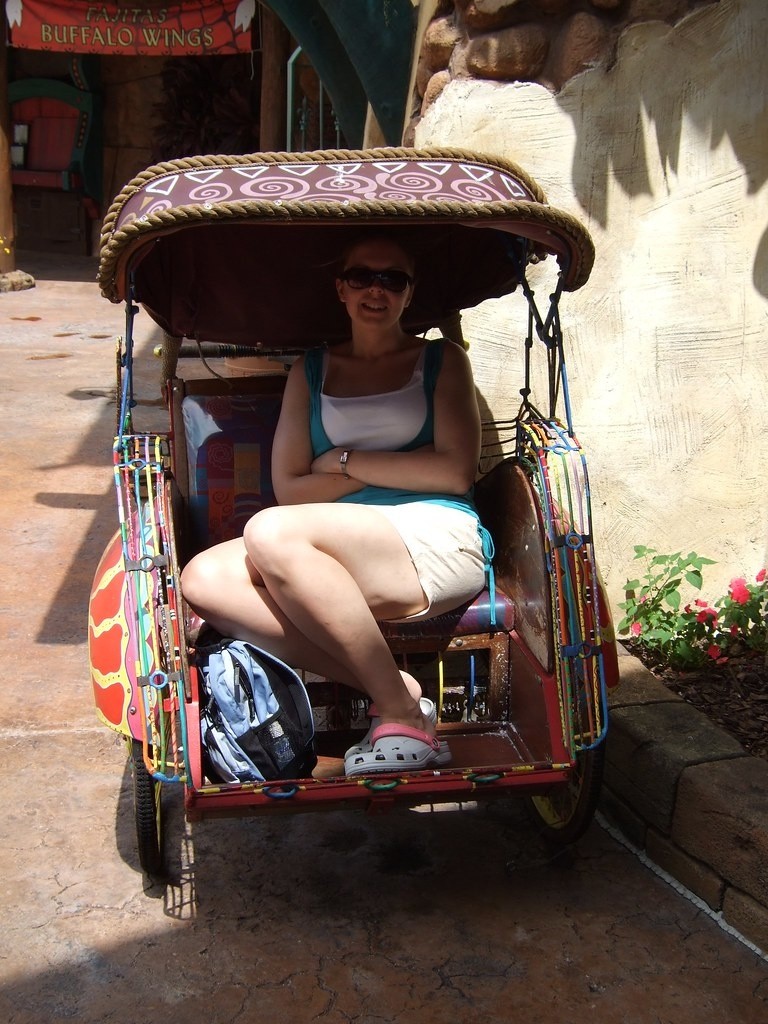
[{"left": 83, "top": 142, "right": 611, "bottom": 873}]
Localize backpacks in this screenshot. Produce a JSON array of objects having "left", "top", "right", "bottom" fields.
[{"left": 195, "top": 637, "right": 316, "bottom": 784}]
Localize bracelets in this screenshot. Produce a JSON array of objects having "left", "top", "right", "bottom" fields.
[{"left": 340, "top": 448, "right": 355, "bottom": 476}]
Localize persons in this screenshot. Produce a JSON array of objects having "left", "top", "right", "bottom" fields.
[{"left": 179, "top": 227, "right": 494, "bottom": 775}]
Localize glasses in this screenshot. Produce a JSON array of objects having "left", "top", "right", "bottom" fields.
[{"left": 341, "top": 265, "right": 415, "bottom": 293}]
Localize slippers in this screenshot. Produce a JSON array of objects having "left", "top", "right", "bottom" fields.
[
  {"left": 345, "top": 723, "right": 453, "bottom": 776},
  {"left": 343, "top": 696, "right": 438, "bottom": 762}
]
